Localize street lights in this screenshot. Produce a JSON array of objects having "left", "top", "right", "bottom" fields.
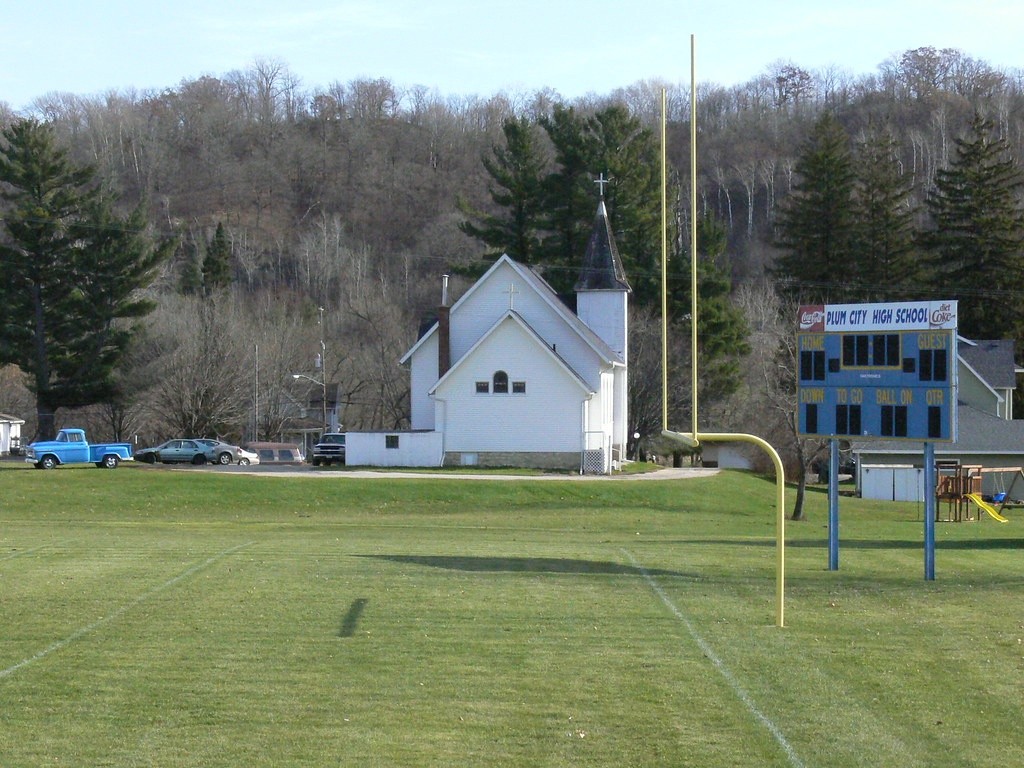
[{"left": 292, "top": 375, "right": 326, "bottom": 435}]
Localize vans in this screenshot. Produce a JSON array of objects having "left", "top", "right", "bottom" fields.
[{"left": 244, "top": 441, "right": 303, "bottom": 466}]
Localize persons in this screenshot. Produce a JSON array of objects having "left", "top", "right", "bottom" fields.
[{"left": 652, "top": 454, "right": 655, "bottom": 463}]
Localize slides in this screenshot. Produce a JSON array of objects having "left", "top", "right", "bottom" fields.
[{"left": 966, "top": 493, "right": 1009, "bottom": 523}]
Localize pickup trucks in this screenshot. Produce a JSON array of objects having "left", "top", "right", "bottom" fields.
[{"left": 24, "top": 428, "right": 134, "bottom": 470}]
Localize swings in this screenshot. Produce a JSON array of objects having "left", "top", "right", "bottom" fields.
[{"left": 992, "top": 472, "right": 1007, "bottom": 502}]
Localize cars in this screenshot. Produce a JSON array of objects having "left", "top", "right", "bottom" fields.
[
  {"left": 193, "top": 439, "right": 260, "bottom": 466},
  {"left": 134, "top": 438, "right": 215, "bottom": 466}
]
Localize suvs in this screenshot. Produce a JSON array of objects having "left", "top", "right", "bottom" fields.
[{"left": 313, "top": 433, "right": 346, "bottom": 466}]
